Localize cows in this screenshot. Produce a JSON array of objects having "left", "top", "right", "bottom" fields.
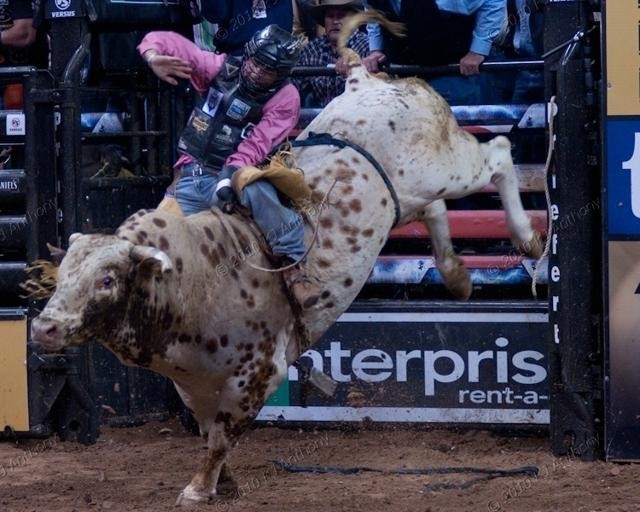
[{"left": 30, "top": 10, "right": 542, "bottom": 508}]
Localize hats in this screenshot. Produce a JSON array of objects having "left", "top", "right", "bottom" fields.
[{"left": 308, "top": 0, "right": 367, "bottom": 29}]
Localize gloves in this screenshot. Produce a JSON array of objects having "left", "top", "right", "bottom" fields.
[{"left": 212, "top": 166, "right": 237, "bottom": 213}]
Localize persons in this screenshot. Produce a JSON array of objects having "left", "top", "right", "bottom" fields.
[
  {"left": 362, "top": 0, "right": 510, "bottom": 105},
  {"left": 288, "top": 0, "right": 370, "bottom": 107},
  {"left": 201, "top": 0, "right": 292, "bottom": 59},
  {"left": 137, "top": 23, "right": 321, "bottom": 308},
  {"left": 509, "top": 0, "right": 576, "bottom": 165},
  {"left": 1, "top": 0, "right": 51, "bottom": 170}
]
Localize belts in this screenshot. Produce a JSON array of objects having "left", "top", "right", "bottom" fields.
[{"left": 178, "top": 168, "right": 219, "bottom": 178}]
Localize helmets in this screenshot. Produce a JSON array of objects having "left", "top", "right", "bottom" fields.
[{"left": 237, "top": 24, "right": 301, "bottom": 103}]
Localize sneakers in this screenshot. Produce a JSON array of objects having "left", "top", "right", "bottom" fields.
[{"left": 281, "top": 260, "right": 322, "bottom": 309}]
